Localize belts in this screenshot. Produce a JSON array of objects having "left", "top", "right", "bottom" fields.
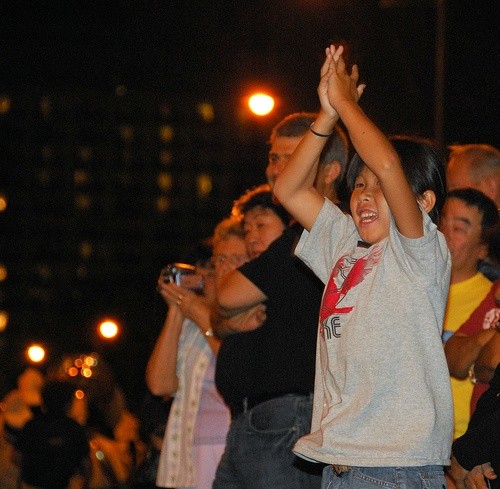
[{"left": 230, "top": 389, "right": 309, "bottom": 417}]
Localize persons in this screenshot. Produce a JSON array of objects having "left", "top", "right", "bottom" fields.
[
  {"left": 438, "top": 141, "right": 500, "bottom": 489},
  {"left": 271, "top": 41, "right": 454, "bottom": 489},
  {"left": 0, "top": 114, "right": 348, "bottom": 489}
]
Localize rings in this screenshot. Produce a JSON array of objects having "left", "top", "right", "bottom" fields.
[
  {"left": 177, "top": 295, "right": 183, "bottom": 301},
  {"left": 176, "top": 300, "right": 181, "bottom": 306}
]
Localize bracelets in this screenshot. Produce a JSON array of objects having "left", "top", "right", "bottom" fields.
[
  {"left": 468, "top": 364, "right": 479, "bottom": 384},
  {"left": 309, "top": 122, "right": 334, "bottom": 137},
  {"left": 203, "top": 327, "right": 214, "bottom": 338}
]
